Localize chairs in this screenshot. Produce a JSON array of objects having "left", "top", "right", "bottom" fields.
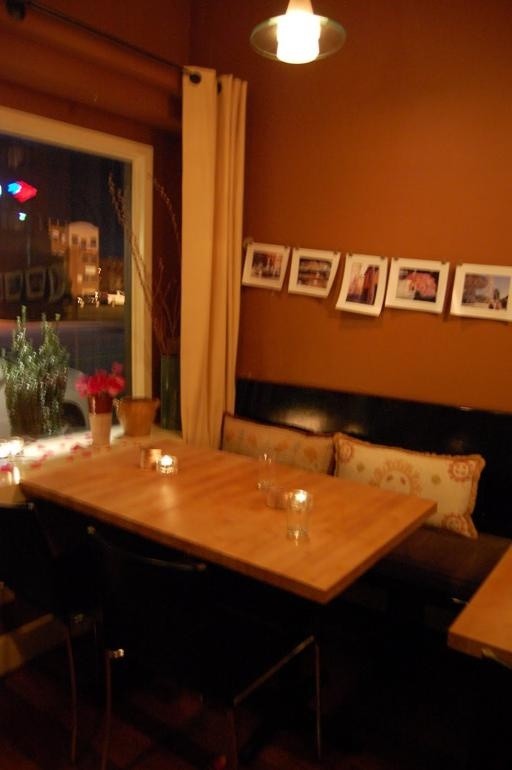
[
  {"left": 1, "top": 566, "right": 109, "bottom": 770},
  {"left": 87, "top": 522, "right": 324, "bottom": 770}
]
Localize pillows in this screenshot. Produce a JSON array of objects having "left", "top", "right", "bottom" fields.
[
  {"left": 218, "top": 410, "right": 339, "bottom": 476},
  {"left": 333, "top": 430, "right": 486, "bottom": 541}
]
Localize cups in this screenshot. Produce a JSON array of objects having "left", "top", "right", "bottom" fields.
[
  {"left": 156, "top": 456, "right": 176, "bottom": 476},
  {"left": 141, "top": 447, "right": 161, "bottom": 471},
  {"left": 1, "top": 435, "right": 26, "bottom": 461},
  {"left": 266, "top": 486, "right": 293, "bottom": 509},
  {"left": 256, "top": 446, "right": 277, "bottom": 488},
  {"left": 284, "top": 488, "right": 312, "bottom": 544}
]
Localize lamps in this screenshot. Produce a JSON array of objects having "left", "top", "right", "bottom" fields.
[{"left": 247, "top": 1, "right": 347, "bottom": 66}]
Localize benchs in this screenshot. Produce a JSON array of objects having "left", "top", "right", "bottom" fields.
[{"left": 222, "top": 377, "right": 512, "bottom": 630}]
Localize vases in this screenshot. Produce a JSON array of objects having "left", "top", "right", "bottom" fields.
[{"left": 86, "top": 395, "right": 115, "bottom": 448}]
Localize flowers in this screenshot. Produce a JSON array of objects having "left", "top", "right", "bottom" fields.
[{"left": 75, "top": 362, "right": 127, "bottom": 399}]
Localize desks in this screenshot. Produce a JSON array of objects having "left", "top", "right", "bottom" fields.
[{"left": 14, "top": 436, "right": 442, "bottom": 682}]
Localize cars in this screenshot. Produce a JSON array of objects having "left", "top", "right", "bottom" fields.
[{"left": 77, "top": 290, "right": 126, "bottom": 308}]
